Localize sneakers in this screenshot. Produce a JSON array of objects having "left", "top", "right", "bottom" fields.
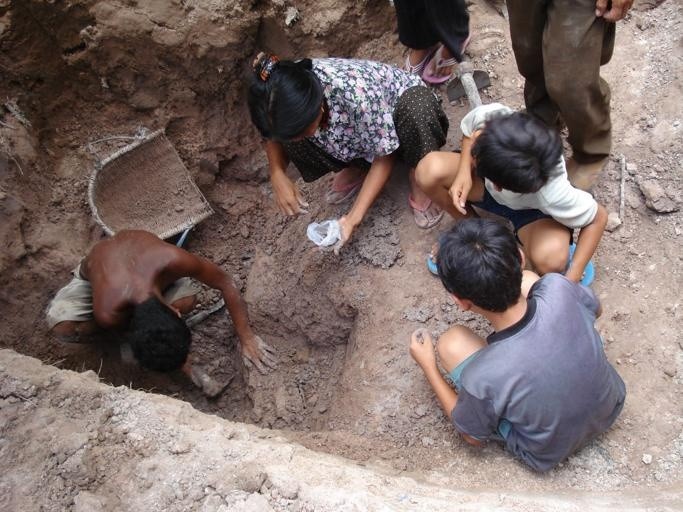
[{"left": 566, "top": 156, "right": 608, "bottom": 191}]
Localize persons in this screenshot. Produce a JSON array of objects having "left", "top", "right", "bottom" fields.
[
  {"left": 393, "top": 1, "right": 474, "bottom": 86},
  {"left": 480, "top": 0, "right": 635, "bottom": 193},
  {"left": 247, "top": 51, "right": 449, "bottom": 258},
  {"left": 43, "top": 228, "right": 278, "bottom": 377},
  {"left": 412, "top": 101, "right": 609, "bottom": 287},
  {"left": 408, "top": 216, "right": 626, "bottom": 473}
]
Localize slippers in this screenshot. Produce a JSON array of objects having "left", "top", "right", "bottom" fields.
[
  {"left": 326, "top": 174, "right": 364, "bottom": 205},
  {"left": 422, "top": 32, "right": 471, "bottom": 83},
  {"left": 409, "top": 191, "right": 443, "bottom": 229},
  {"left": 561, "top": 242, "right": 594, "bottom": 286},
  {"left": 402, "top": 44, "right": 438, "bottom": 79},
  {"left": 428, "top": 234, "right": 452, "bottom": 274}
]
